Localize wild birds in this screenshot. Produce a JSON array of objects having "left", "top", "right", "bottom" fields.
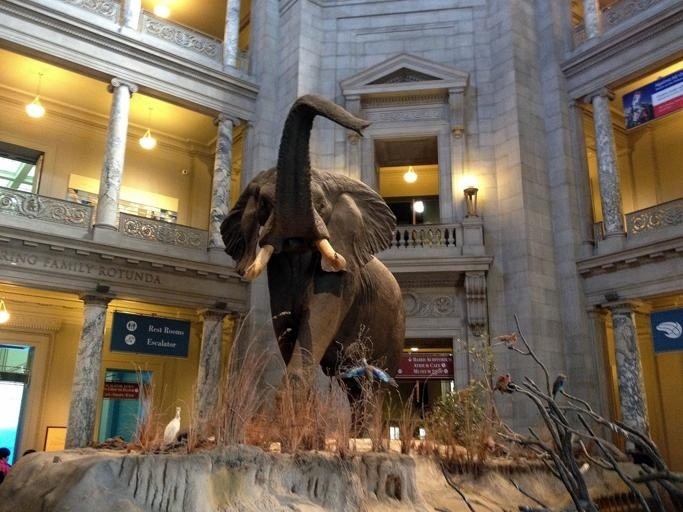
[
  {"left": 341, "top": 358, "right": 400, "bottom": 388},
  {"left": 553, "top": 374, "right": 567, "bottom": 398},
  {"left": 496, "top": 372, "right": 511, "bottom": 395},
  {"left": 163, "top": 406, "right": 181, "bottom": 452},
  {"left": 493, "top": 331, "right": 518, "bottom": 350}
]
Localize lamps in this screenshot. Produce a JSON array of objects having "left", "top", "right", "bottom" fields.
[
  {"left": 24, "top": 72, "right": 47, "bottom": 119},
  {"left": 402, "top": 166, "right": 418, "bottom": 184},
  {"left": 460, "top": 174, "right": 479, "bottom": 216},
  {"left": 138, "top": 108, "right": 157, "bottom": 151}
]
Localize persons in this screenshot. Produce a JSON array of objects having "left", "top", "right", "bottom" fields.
[{"left": 0, "top": 447, "right": 11, "bottom": 485}]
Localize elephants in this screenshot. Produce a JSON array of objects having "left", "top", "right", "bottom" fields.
[{"left": 219, "top": 93, "right": 407, "bottom": 442}]
